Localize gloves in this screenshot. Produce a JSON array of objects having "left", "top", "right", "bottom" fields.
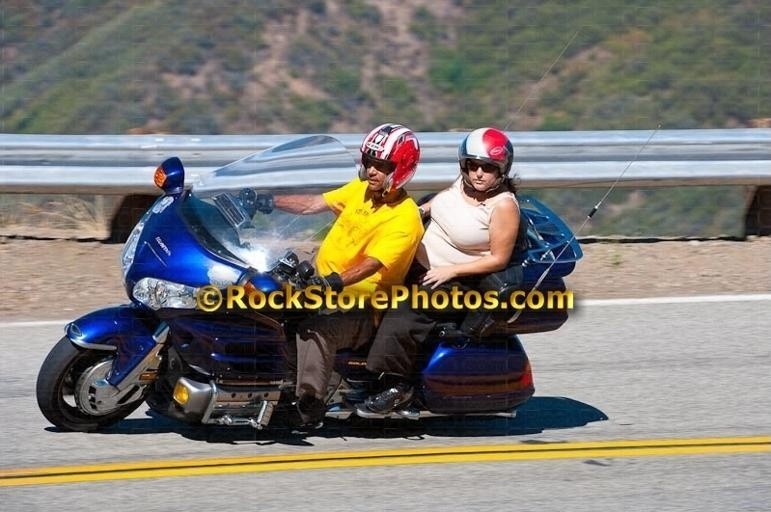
[
  {"left": 239, "top": 187, "right": 274, "bottom": 214},
  {"left": 300, "top": 270, "right": 348, "bottom": 300}
]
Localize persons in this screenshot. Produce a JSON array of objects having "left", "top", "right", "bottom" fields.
[
  {"left": 241, "top": 122, "right": 426, "bottom": 424},
  {"left": 364, "top": 127, "right": 521, "bottom": 413}
]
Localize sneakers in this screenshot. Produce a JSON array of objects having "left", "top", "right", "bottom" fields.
[
  {"left": 365, "top": 382, "right": 416, "bottom": 415},
  {"left": 273, "top": 403, "right": 325, "bottom": 431}
]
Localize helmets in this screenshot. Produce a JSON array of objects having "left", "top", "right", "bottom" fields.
[
  {"left": 457, "top": 126, "right": 514, "bottom": 196},
  {"left": 358, "top": 121, "right": 421, "bottom": 196}
]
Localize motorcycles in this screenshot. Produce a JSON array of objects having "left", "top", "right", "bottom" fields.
[{"left": 33, "top": 134, "right": 584, "bottom": 432}]
